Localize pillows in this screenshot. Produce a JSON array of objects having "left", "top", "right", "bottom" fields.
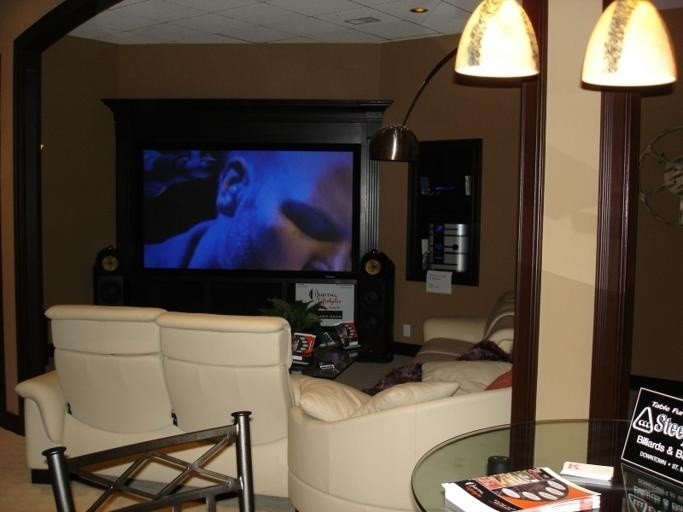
[
  {"left": 422, "top": 360, "right": 511, "bottom": 396},
  {"left": 298, "top": 376, "right": 368, "bottom": 423},
  {"left": 350, "top": 381, "right": 462, "bottom": 417}
]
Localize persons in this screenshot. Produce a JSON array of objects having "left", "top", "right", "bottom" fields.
[{"left": 144, "top": 150, "right": 353, "bottom": 271}]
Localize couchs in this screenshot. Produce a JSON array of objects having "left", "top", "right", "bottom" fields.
[
  {"left": 287, "top": 292, "right": 514, "bottom": 512},
  {"left": 17, "top": 304, "right": 350, "bottom": 498}
]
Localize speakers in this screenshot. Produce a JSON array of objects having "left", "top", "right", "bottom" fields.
[
  {"left": 92, "top": 245, "right": 126, "bottom": 307},
  {"left": 356, "top": 249, "right": 398, "bottom": 362}
]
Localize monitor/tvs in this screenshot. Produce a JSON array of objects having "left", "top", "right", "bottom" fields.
[{"left": 108, "top": 96, "right": 395, "bottom": 293}]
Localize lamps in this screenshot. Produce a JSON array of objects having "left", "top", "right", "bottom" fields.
[
  {"left": 454, "top": 1, "right": 541, "bottom": 80},
  {"left": 583, "top": 1, "right": 679, "bottom": 90},
  {"left": 368, "top": 47, "right": 456, "bottom": 161}
]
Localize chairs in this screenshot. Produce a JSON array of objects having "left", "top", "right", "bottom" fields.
[{"left": 43, "top": 406, "right": 255, "bottom": 512}]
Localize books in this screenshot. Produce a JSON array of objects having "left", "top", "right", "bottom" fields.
[{"left": 441, "top": 467, "right": 602, "bottom": 512}]
